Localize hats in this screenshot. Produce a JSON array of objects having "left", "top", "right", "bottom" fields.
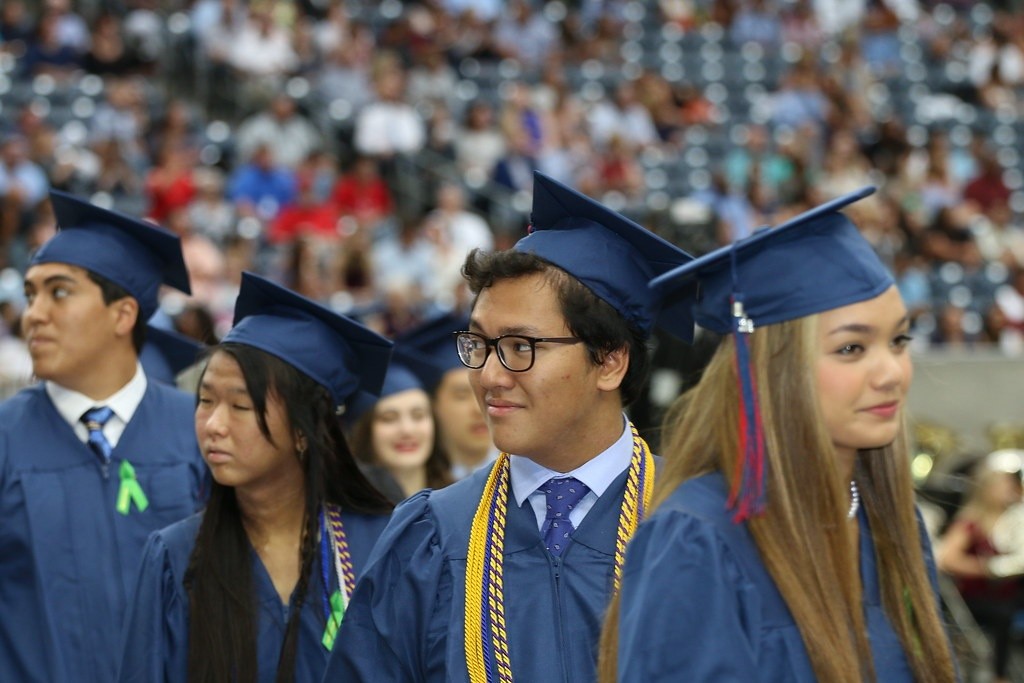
[
  {"left": 142, "top": 324, "right": 211, "bottom": 385},
  {"left": 513, "top": 170, "right": 700, "bottom": 350},
  {"left": 220, "top": 272, "right": 396, "bottom": 421},
  {"left": 646, "top": 188, "right": 897, "bottom": 520},
  {"left": 21, "top": 189, "right": 195, "bottom": 322},
  {"left": 347, "top": 303, "right": 474, "bottom": 399}
]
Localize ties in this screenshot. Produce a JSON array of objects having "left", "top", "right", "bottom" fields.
[
  {"left": 536, "top": 476, "right": 588, "bottom": 562},
  {"left": 81, "top": 407, "right": 113, "bottom": 464}
]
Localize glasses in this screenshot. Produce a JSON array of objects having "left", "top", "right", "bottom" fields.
[{"left": 453, "top": 329, "right": 586, "bottom": 372}]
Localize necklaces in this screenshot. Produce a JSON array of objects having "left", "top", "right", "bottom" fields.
[{"left": 847, "top": 480, "right": 860, "bottom": 518}]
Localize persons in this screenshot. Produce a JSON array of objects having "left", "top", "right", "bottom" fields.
[
  {"left": 0, "top": 189, "right": 211, "bottom": 683},
  {"left": 0, "top": 0, "right": 1024, "bottom": 497},
  {"left": 931, "top": 447, "right": 1024, "bottom": 683},
  {"left": 618, "top": 186, "right": 965, "bottom": 683},
  {"left": 324, "top": 170, "right": 696, "bottom": 683},
  {"left": 115, "top": 271, "right": 395, "bottom": 683}
]
